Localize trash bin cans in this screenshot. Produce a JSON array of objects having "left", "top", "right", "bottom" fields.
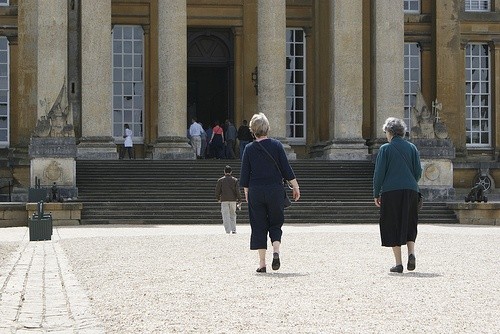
[{"left": 28, "top": 200, "right": 53, "bottom": 241}]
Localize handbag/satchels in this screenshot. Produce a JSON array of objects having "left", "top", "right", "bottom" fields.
[{"left": 281, "top": 178, "right": 293, "bottom": 208}]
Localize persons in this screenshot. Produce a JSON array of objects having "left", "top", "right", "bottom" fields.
[
  {"left": 216, "top": 166, "right": 241, "bottom": 234},
  {"left": 121, "top": 124, "right": 134, "bottom": 159},
  {"left": 187, "top": 116, "right": 236, "bottom": 160},
  {"left": 238, "top": 120, "right": 251, "bottom": 160},
  {"left": 240, "top": 113, "right": 300, "bottom": 272},
  {"left": 373, "top": 118, "right": 422, "bottom": 273}
]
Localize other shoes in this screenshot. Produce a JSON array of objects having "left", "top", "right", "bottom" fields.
[
  {"left": 390, "top": 265, "right": 404, "bottom": 273},
  {"left": 232, "top": 231, "right": 236, "bottom": 234},
  {"left": 256, "top": 267, "right": 266, "bottom": 272},
  {"left": 407, "top": 254, "right": 416, "bottom": 271},
  {"left": 271, "top": 252, "right": 280, "bottom": 270}
]
